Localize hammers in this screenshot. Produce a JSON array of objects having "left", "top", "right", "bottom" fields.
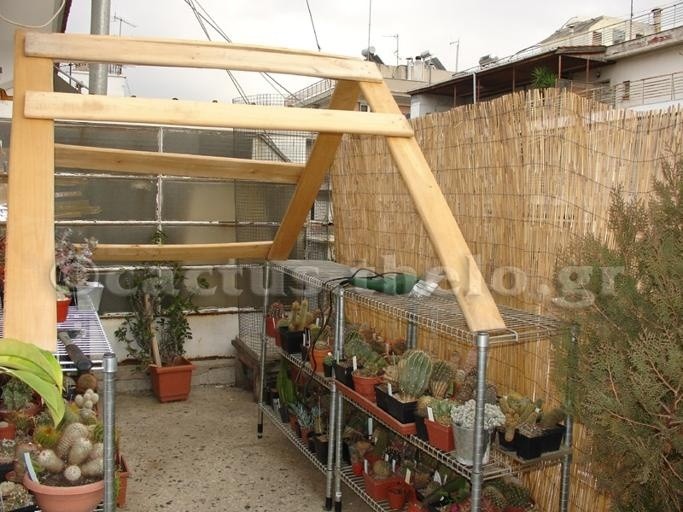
[{"left": 57, "top": 328, "right": 92, "bottom": 372}]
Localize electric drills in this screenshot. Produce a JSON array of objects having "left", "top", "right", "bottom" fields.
[{"left": 349, "top": 273, "right": 423, "bottom": 294}]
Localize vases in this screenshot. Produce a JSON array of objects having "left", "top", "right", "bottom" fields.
[{"left": 56, "top": 294, "right": 71, "bottom": 324}]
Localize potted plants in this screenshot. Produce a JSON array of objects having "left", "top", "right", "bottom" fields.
[
  {"left": 267, "top": 297, "right": 332, "bottom": 466},
  {"left": 1, "top": 360, "right": 131, "bottom": 512},
  {"left": 333, "top": 326, "right": 570, "bottom": 512},
  {"left": 113, "top": 225, "right": 196, "bottom": 404}
]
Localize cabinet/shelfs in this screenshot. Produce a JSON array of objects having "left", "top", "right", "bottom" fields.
[
  {"left": 257, "top": 255, "right": 342, "bottom": 512},
  {"left": 305, "top": 181, "right": 335, "bottom": 263},
  {"left": 335, "top": 275, "right": 577, "bottom": 512}
]
